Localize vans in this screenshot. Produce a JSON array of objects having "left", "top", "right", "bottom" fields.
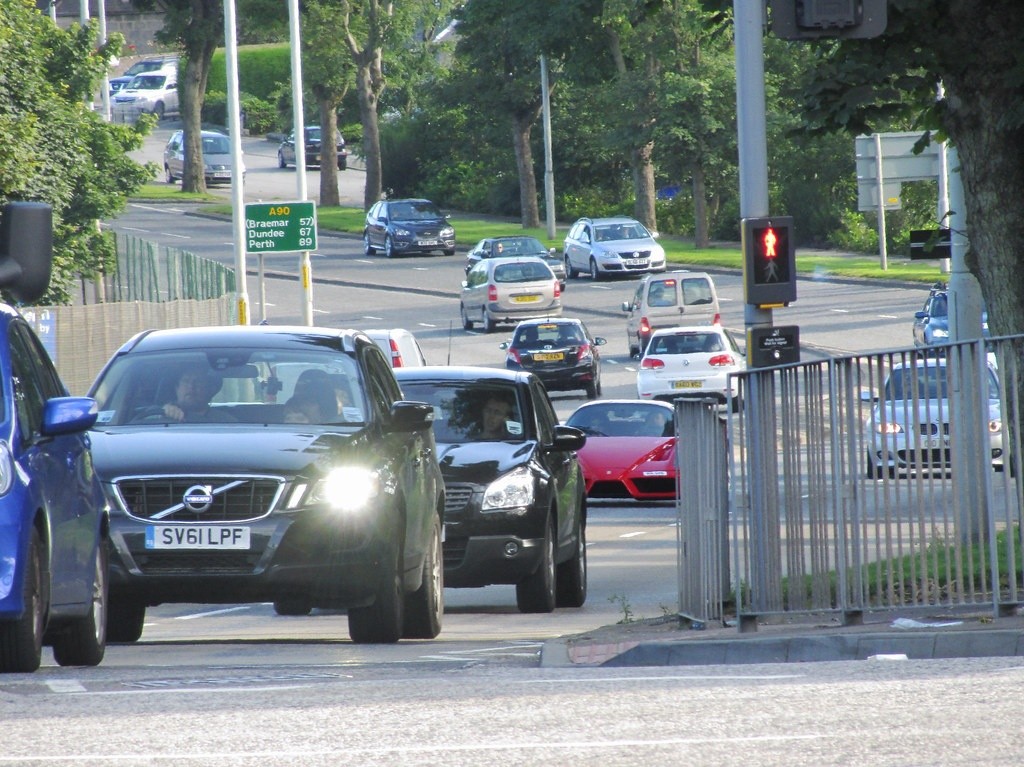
[
  {"left": 460, "top": 257, "right": 562, "bottom": 331},
  {"left": 622, "top": 267, "right": 722, "bottom": 356},
  {"left": 268, "top": 327, "right": 424, "bottom": 405}
]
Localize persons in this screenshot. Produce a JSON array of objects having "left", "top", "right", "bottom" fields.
[
  {"left": 597, "top": 231, "right": 608, "bottom": 241},
  {"left": 586, "top": 411, "right": 612, "bottom": 436},
  {"left": 621, "top": 227, "right": 633, "bottom": 238},
  {"left": 497, "top": 242, "right": 508, "bottom": 257},
  {"left": 144, "top": 366, "right": 238, "bottom": 422},
  {"left": 280, "top": 368, "right": 344, "bottom": 423},
  {"left": 472, "top": 394, "right": 512, "bottom": 440},
  {"left": 635, "top": 411, "right": 669, "bottom": 436},
  {"left": 651, "top": 288, "right": 672, "bottom": 306},
  {"left": 704, "top": 334, "right": 722, "bottom": 351}
]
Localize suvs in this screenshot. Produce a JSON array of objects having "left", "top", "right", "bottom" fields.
[
  {"left": 109, "top": 54, "right": 179, "bottom": 95},
  {"left": 111, "top": 69, "right": 181, "bottom": 122}
]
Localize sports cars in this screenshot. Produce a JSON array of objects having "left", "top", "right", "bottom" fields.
[{"left": 560, "top": 401, "right": 680, "bottom": 499}]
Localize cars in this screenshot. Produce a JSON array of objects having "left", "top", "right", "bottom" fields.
[
  {"left": 501, "top": 317, "right": 607, "bottom": 396},
  {"left": 0, "top": 301, "right": 113, "bottom": 668},
  {"left": 166, "top": 132, "right": 247, "bottom": 184},
  {"left": 362, "top": 198, "right": 457, "bottom": 256},
  {"left": 278, "top": 126, "right": 348, "bottom": 169},
  {"left": 82, "top": 327, "right": 447, "bottom": 644},
  {"left": 635, "top": 327, "right": 750, "bottom": 409},
  {"left": 388, "top": 363, "right": 589, "bottom": 612},
  {"left": 562, "top": 217, "right": 668, "bottom": 281},
  {"left": 914, "top": 288, "right": 996, "bottom": 355},
  {"left": 860, "top": 361, "right": 1006, "bottom": 476},
  {"left": 469, "top": 235, "right": 568, "bottom": 289}
]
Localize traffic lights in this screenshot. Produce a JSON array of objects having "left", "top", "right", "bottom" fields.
[{"left": 741, "top": 216, "right": 797, "bottom": 307}]
